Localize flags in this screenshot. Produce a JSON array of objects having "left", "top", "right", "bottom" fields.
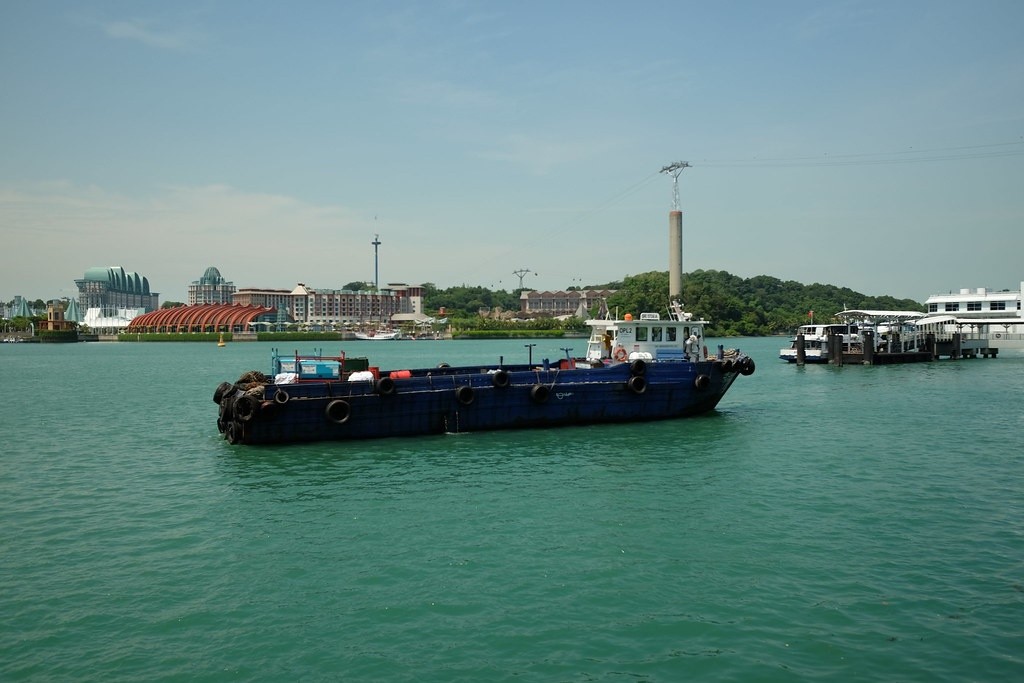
[
  {"left": 596, "top": 301, "right": 607, "bottom": 319},
  {"left": 807, "top": 311, "right": 812, "bottom": 317}
]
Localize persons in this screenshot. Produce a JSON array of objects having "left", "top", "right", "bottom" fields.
[{"left": 687, "top": 334, "right": 701, "bottom": 355}]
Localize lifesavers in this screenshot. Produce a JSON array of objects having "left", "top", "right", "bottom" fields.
[{"left": 615, "top": 348, "right": 627, "bottom": 361}]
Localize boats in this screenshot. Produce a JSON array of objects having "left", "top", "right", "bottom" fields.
[
  {"left": 211, "top": 297, "right": 754, "bottom": 446},
  {"left": 779, "top": 310, "right": 887, "bottom": 364},
  {"left": 353, "top": 324, "right": 402, "bottom": 341}
]
